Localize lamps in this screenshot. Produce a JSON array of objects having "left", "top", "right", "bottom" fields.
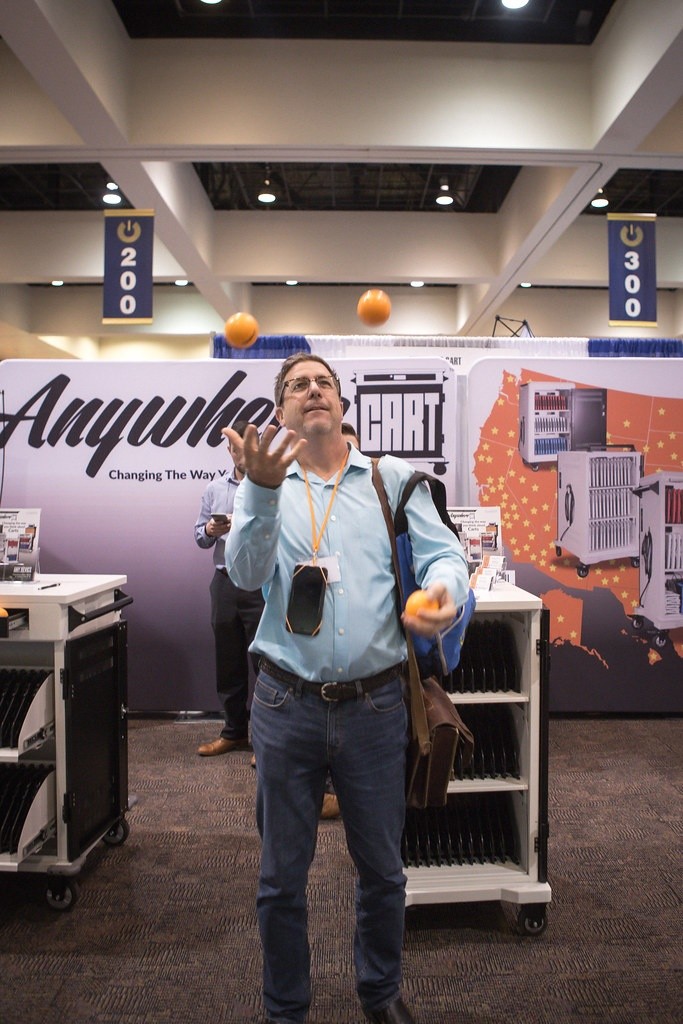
[{"left": 100, "top": 172, "right": 608, "bottom": 211}]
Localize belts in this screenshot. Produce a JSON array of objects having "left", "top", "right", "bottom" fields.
[{"left": 260, "top": 657, "right": 403, "bottom": 701}]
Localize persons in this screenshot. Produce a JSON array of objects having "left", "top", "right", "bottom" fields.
[
  {"left": 194, "top": 421, "right": 252, "bottom": 767},
  {"left": 226, "top": 353, "right": 470, "bottom": 1024}
]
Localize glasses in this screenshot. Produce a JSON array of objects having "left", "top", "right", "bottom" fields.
[{"left": 279, "top": 375, "right": 341, "bottom": 406}]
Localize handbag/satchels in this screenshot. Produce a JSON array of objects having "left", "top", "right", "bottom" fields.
[
  {"left": 405, "top": 676, "right": 474, "bottom": 809},
  {"left": 395, "top": 470, "right": 476, "bottom": 676}
]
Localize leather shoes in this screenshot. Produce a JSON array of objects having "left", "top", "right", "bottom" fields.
[
  {"left": 251, "top": 754, "right": 256, "bottom": 765},
  {"left": 362, "top": 997, "right": 414, "bottom": 1024},
  {"left": 197, "top": 737, "right": 248, "bottom": 755}
]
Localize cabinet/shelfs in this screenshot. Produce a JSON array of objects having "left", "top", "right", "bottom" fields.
[
  {"left": 401, "top": 577, "right": 552, "bottom": 937},
  {"left": 0, "top": 570, "right": 134, "bottom": 912},
  {"left": 515, "top": 382, "right": 683, "bottom": 646}
]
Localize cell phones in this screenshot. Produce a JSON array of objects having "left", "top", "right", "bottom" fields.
[
  {"left": 211, "top": 512, "right": 230, "bottom": 525},
  {"left": 285, "top": 564, "right": 327, "bottom": 636}
]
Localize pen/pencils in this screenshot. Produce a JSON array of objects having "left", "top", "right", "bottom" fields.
[{"left": 36, "top": 582, "right": 61, "bottom": 591}]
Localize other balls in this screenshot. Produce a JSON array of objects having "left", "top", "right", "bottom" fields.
[
  {"left": 405, "top": 589, "right": 440, "bottom": 620},
  {"left": 355, "top": 289, "right": 391, "bottom": 327},
  {"left": 224, "top": 312, "right": 260, "bottom": 350}
]
